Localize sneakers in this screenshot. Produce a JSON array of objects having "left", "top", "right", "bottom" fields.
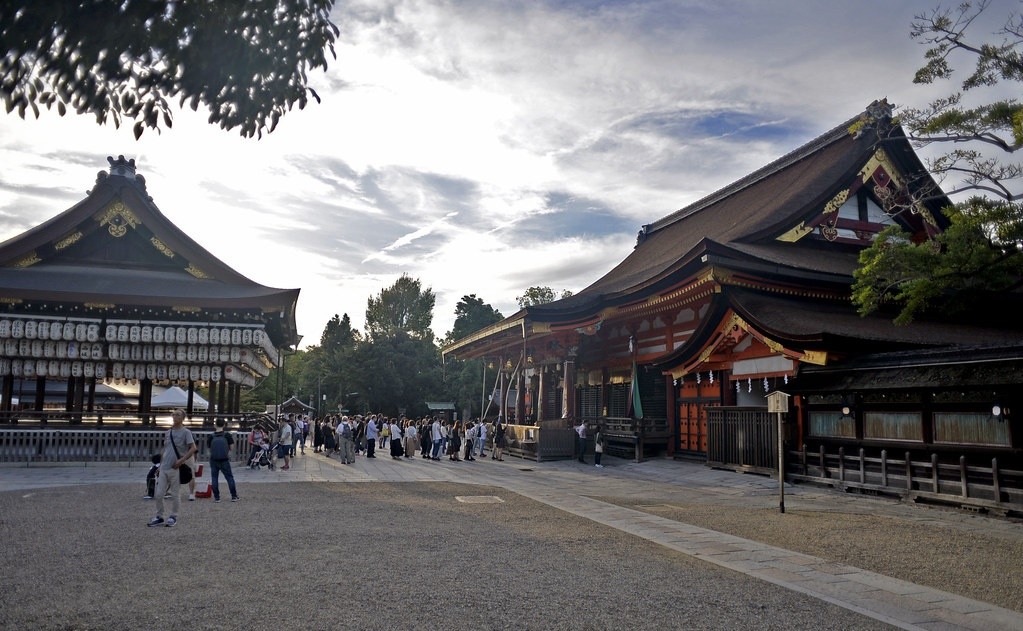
[
  {"left": 166, "top": 517, "right": 177, "bottom": 527},
  {"left": 147, "top": 516, "right": 165, "bottom": 526}
]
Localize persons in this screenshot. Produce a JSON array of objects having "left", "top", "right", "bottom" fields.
[
  {"left": 146, "top": 409, "right": 196, "bottom": 528},
  {"left": 576, "top": 420, "right": 589, "bottom": 465},
  {"left": 595, "top": 426, "right": 604, "bottom": 469},
  {"left": 206, "top": 417, "right": 241, "bottom": 503},
  {"left": 287, "top": 412, "right": 490, "bottom": 465},
  {"left": 491, "top": 421, "right": 508, "bottom": 461},
  {"left": 246, "top": 424, "right": 271, "bottom": 470},
  {"left": 278, "top": 418, "right": 292, "bottom": 471},
  {"left": 143, "top": 438, "right": 198, "bottom": 501}
]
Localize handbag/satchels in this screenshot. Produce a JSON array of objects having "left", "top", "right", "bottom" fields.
[
  {"left": 382, "top": 425, "right": 389, "bottom": 437},
  {"left": 179, "top": 463, "right": 193, "bottom": 484},
  {"left": 595, "top": 444, "right": 602, "bottom": 452},
  {"left": 446, "top": 446, "right": 454, "bottom": 455}
]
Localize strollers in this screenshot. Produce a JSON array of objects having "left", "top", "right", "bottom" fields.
[{"left": 248, "top": 441, "right": 281, "bottom": 471}]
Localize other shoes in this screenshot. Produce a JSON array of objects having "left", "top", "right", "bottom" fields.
[
  {"left": 580, "top": 461, "right": 588, "bottom": 464},
  {"left": 214, "top": 498, "right": 221, "bottom": 503},
  {"left": 281, "top": 465, "right": 289, "bottom": 470},
  {"left": 288, "top": 451, "right": 504, "bottom": 464},
  {"left": 189, "top": 494, "right": 196, "bottom": 500},
  {"left": 595, "top": 464, "right": 604, "bottom": 468},
  {"left": 230, "top": 496, "right": 240, "bottom": 501},
  {"left": 246, "top": 466, "right": 250, "bottom": 469}
]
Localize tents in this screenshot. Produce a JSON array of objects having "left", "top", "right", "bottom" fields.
[{"left": 151, "top": 386, "right": 209, "bottom": 411}]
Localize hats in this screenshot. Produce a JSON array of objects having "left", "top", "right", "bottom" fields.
[
  {"left": 355, "top": 414, "right": 363, "bottom": 419},
  {"left": 253, "top": 424, "right": 262, "bottom": 430},
  {"left": 342, "top": 416, "right": 348, "bottom": 421}
]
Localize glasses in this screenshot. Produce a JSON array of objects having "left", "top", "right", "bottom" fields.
[{"left": 170, "top": 413, "right": 183, "bottom": 417}]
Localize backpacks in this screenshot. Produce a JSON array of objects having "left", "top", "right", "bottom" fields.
[
  {"left": 491, "top": 426, "right": 497, "bottom": 438},
  {"left": 212, "top": 431, "right": 229, "bottom": 460},
  {"left": 342, "top": 423, "right": 352, "bottom": 439}
]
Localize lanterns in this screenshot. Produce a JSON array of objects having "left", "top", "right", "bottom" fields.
[
  {"left": 258, "top": 333, "right": 278, "bottom": 369},
  {"left": 105, "top": 324, "right": 263, "bottom": 346},
  {"left": 113, "top": 363, "right": 222, "bottom": 382},
  {"left": 224, "top": 364, "right": 255, "bottom": 388},
  {"left": 108, "top": 344, "right": 242, "bottom": 363},
  {"left": 0, "top": 340, "right": 104, "bottom": 360},
  {"left": 0, "top": 319, "right": 101, "bottom": 342},
  {"left": 240, "top": 348, "right": 269, "bottom": 377},
  {"left": 0, "top": 360, "right": 107, "bottom": 378},
  {"left": 323, "top": 394, "right": 326, "bottom": 401}
]
